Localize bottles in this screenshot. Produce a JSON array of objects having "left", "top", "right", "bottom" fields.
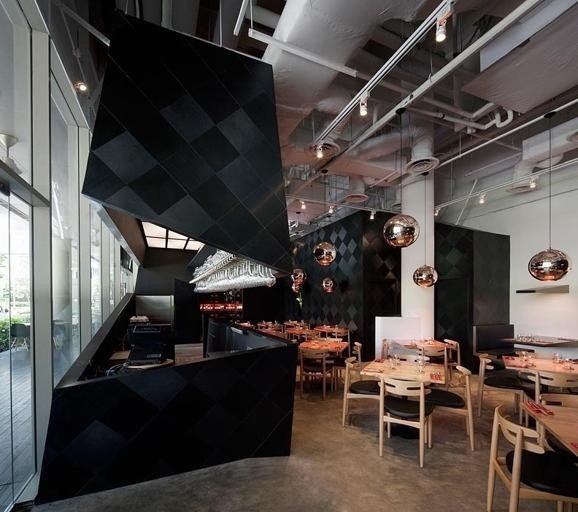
[{"left": 519, "top": 351, "right": 536, "bottom": 364}]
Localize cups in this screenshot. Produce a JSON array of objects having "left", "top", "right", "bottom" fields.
[
  {"left": 553, "top": 352, "right": 573, "bottom": 370},
  {"left": 388, "top": 352, "right": 401, "bottom": 370},
  {"left": 417, "top": 354, "right": 428, "bottom": 375},
  {"left": 517, "top": 333, "right": 540, "bottom": 343}
]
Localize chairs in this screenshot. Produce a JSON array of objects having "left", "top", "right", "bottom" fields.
[
  {"left": 342, "top": 321, "right": 577, "bottom": 511},
  {"left": 10, "top": 321, "right": 64, "bottom": 352},
  {"left": 238, "top": 320, "right": 351, "bottom": 402}
]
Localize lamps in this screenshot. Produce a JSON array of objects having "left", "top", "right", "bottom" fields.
[
  {"left": 286, "top": 194, "right": 401, "bottom": 220},
  {"left": 312, "top": 0, "right": 456, "bottom": 159},
  {"left": 434, "top": 158, "right": 578, "bottom": 216}
]
